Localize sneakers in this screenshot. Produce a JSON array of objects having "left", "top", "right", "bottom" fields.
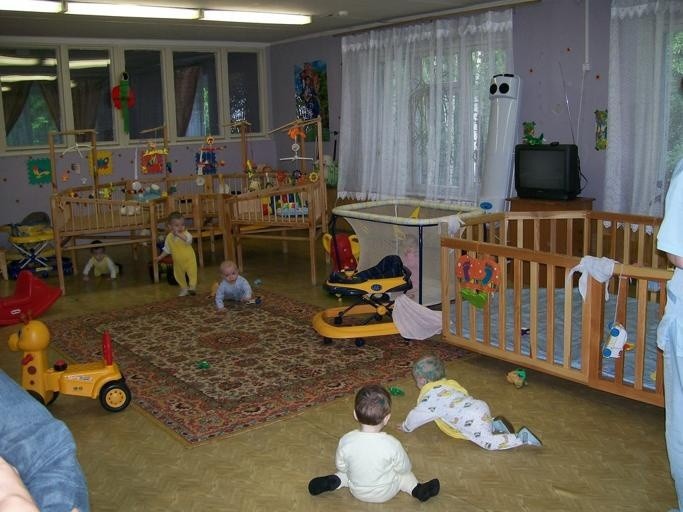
[
  {"left": 178, "top": 289, "right": 189, "bottom": 297},
  {"left": 491, "top": 415, "right": 515, "bottom": 434},
  {"left": 515, "top": 424, "right": 543, "bottom": 448},
  {"left": 187, "top": 288, "right": 196, "bottom": 296},
  {"left": 308, "top": 474, "right": 341, "bottom": 496},
  {"left": 412, "top": 478, "right": 440, "bottom": 503}
]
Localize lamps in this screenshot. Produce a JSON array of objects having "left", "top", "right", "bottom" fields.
[{"left": 0, "top": 1, "right": 312, "bottom": 27}]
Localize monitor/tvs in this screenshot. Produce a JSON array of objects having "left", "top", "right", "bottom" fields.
[{"left": 515, "top": 144, "right": 580, "bottom": 200}]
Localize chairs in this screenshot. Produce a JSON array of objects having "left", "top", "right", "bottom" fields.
[{"left": 0, "top": 223, "right": 55, "bottom": 282}]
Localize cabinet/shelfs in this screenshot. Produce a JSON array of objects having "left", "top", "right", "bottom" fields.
[{"left": 499, "top": 194, "right": 595, "bottom": 292}]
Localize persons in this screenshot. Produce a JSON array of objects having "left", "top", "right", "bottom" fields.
[
  {"left": 211, "top": 259, "right": 253, "bottom": 313},
  {"left": 307, "top": 384, "right": 440, "bottom": 502},
  {"left": 654, "top": 157, "right": 683, "bottom": 512},
  {"left": 80, "top": 239, "right": 123, "bottom": 283},
  {"left": 153, "top": 212, "right": 198, "bottom": 297},
  {"left": 0, "top": 368, "right": 92, "bottom": 511},
  {"left": 397, "top": 354, "right": 543, "bottom": 450}
]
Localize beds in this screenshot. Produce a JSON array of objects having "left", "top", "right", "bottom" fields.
[
  {"left": 50, "top": 116, "right": 329, "bottom": 295},
  {"left": 432, "top": 207, "right": 676, "bottom": 414}
]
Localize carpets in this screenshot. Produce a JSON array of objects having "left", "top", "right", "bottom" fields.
[{"left": 27, "top": 286, "right": 492, "bottom": 451}]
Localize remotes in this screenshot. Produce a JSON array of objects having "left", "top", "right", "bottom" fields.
[{"left": 551, "top": 141, "right": 559, "bottom": 145}]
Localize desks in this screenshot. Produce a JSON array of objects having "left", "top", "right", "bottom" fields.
[{"left": 7, "top": 230, "right": 54, "bottom": 269}]
